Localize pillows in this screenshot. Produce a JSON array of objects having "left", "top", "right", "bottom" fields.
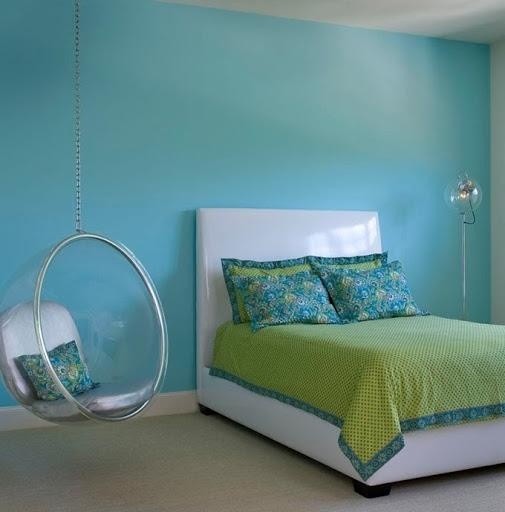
[
  {"left": 213, "top": 250, "right": 431, "bottom": 334},
  {"left": 14, "top": 338, "right": 102, "bottom": 400}
]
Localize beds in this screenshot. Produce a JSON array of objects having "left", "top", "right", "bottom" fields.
[{"left": 189, "top": 208, "right": 505, "bottom": 497}]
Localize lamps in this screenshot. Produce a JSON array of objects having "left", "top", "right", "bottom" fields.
[{"left": 442, "top": 168, "right": 482, "bottom": 321}]
